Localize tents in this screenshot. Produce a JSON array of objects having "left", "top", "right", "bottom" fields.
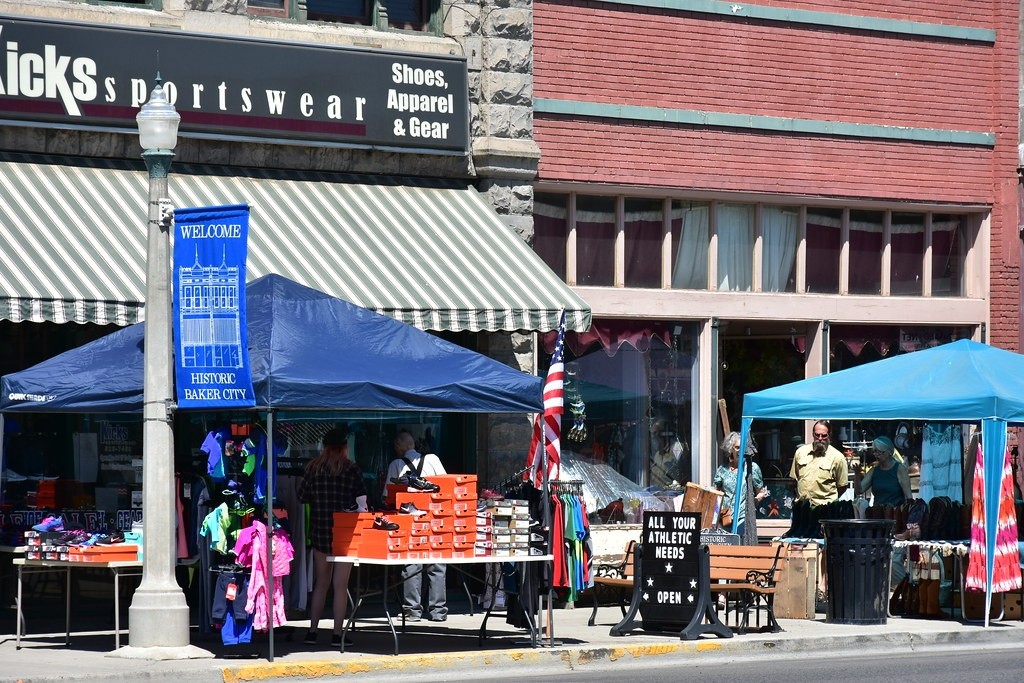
[{"left": 731, "top": 337, "right": 1024, "bottom": 627}]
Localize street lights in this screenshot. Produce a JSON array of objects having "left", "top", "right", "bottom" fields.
[{"left": 106, "top": 46, "right": 216, "bottom": 660}]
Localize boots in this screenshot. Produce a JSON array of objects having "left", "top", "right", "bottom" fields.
[
  {"left": 889, "top": 572, "right": 951, "bottom": 620},
  {"left": 782, "top": 497, "right": 971, "bottom": 539},
  {"left": 363, "top": 478, "right": 397, "bottom": 513}
]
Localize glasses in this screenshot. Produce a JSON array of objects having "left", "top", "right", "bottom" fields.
[
  {"left": 872, "top": 450, "right": 885, "bottom": 454},
  {"left": 814, "top": 433, "right": 828, "bottom": 437}
]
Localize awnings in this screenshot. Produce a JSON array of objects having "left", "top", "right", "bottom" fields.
[{"left": 0, "top": 150, "right": 591, "bottom": 332}]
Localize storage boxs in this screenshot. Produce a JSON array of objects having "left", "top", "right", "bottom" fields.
[
  {"left": 231, "top": 423, "right": 252, "bottom": 436},
  {"left": 331, "top": 474, "right": 528, "bottom": 555},
  {"left": 24, "top": 459, "right": 143, "bottom": 562},
  {"left": 962, "top": 591, "right": 1022, "bottom": 620}
]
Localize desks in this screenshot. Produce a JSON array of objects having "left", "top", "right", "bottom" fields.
[
  {"left": 325, "top": 554, "right": 555, "bottom": 656},
  {"left": 12, "top": 557, "right": 144, "bottom": 652},
  {"left": 764, "top": 535, "right": 1006, "bottom": 624},
  {"left": 0, "top": 545, "right": 28, "bottom": 636}
]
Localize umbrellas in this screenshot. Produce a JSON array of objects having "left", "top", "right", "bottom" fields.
[
  {"left": 523, "top": 312, "right": 565, "bottom": 491},
  {"left": 0, "top": 272, "right": 555, "bottom": 663}
]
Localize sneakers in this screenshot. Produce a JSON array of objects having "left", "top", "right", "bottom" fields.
[
  {"left": 31, "top": 516, "right": 64, "bottom": 532},
  {"left": 51, "top": 530, "right": 125, "bottom": 546},
  {"left": 343, "top": 501, "right": 359, "bottom": 512},
  {"left": 407, "top": 477, "right": 440, "bottom": 493},
  {"left": 400, "top": 501, "right": 428, "bottom": 516},
  {"left": 893, "top": 523, "right": 921, "bottom": 540},
  {"left": 477, "top": 501, "right": 487, "bottom": 512},
  {"left": 390, "top": 472, "right": 417, "bottom": 485},
  {"left": 373, "top": 517, "right": 399, "bottom": 530},
  {"left": 480, "top": 488, "right": 504, "bottom": 501}
]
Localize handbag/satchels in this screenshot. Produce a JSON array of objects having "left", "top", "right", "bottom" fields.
[{"left": 720, "top": 508, "right": 733, "bottom": 526}]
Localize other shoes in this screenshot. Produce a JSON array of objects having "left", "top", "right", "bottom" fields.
[
  {"left": 530, "top": 519, "right": 539, "bottom": 527},
  {"left": 331, "top": 635, "right": 354, "bottom": 646},
  {"left": 815, "top": 587, "right": 828, "bottom": 604},
  {"left": 432, "top": 615, "right": 447, "bottom": 621},
  {"left": 530, "top": 533, "right": 545, "bottom": 541},
  {"left": 718, "top": 594, "right": 725, "bottom": 608},
  {"left": 530, "top": 547, "right": 544, "bottom": 556},
  {"left": 304, "top": 628, "right": 318, "bottom": 643},
  {"left": 397, "top": 613, "right": 420, "bottom": 621}
]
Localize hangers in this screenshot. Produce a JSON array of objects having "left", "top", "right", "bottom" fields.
[
  {"left": 209, "top": 561, "right": 252, "bottom": 575},
  {"left": 492, "top": 465, "right": 583, "bottom": 496}
]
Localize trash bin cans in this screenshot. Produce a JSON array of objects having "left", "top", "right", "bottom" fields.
[{"left": 819, "top": 519, "right": 895, "bottom": 626}]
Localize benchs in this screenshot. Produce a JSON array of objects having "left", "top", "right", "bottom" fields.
[{"left": 586, "top": 540, "right": 788, "bottom": 635}]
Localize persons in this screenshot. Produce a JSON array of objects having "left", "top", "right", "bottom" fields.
[
  {"left": 789, "top": 420, "right": 849, "bottom": 604},
  {"left": 297, "top": 428, "right": 369, "bottom": 646},
  {"left": 851, "top": 436, "right": 913, "bottom": 598},
  {"left": 381, "top": 430, "right": 448, "bottom": 622},
  {"left": 713, "top": 430, "right": 764, "bottom": 608}
]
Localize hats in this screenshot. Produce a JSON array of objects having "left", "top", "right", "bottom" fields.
[{"left": 323, "top": 430, "right": 347, "bottom": 446}]
